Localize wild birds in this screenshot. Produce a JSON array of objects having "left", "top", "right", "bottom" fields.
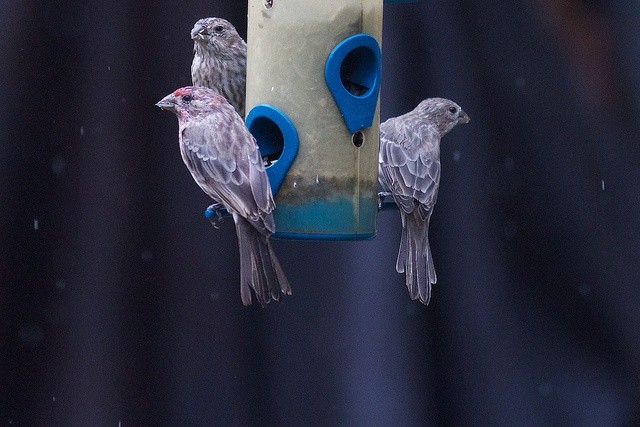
[
  {"left": 189, "top": 16, "right": 247, "bottom": 122},
  {"left": 154, "top": 85, "right": 293, "bottom": 310},
  {"left": 377, "top": 97, "right": 471, "bottom": 307}
]
